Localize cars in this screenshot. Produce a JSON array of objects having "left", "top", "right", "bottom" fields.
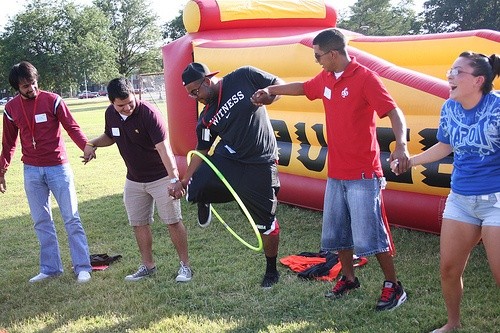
[
  {"left": 0, "top": 97, "right": 13, "bottom": 105},
  {"left": 78, "top": 88, "right": 156, "bottom": 99}
]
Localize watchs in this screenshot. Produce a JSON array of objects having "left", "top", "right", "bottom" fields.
[{"left": 170, "top": 178, "right": 179, "bottom": 183}]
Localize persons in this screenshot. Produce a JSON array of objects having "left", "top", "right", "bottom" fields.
[
  {"left": 0, "top": 60, "right": 99, "bottom": 283},
  {"left": 387, "top": 52, "right": 500, "bottom": 333},
  {"left": 170, "top": 59, "right": 291, "bottom": 290},
  {"left": 82, "top": 76, "right": 195, "bottom": 280},
  {"left": 250, "top": 29, "right": 417, "bottom": 312}
]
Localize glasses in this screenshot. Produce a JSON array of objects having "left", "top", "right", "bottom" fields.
[
  {"left": 445, "top": 69, "right": 475, "bottom": 77},
  {"left": 314, "top": 50, "right": 334, "bottom": 60},
  {"left": 188, "top": 79, "right": 205, "bottom": 98}
]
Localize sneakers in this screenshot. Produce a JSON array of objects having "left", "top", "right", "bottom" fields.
[
  {"left": 375, "top": 280, "right": 407, "bottom": 313},
  {"left": 29, "top": 272, "right": 49, "bottom": 284},
  {"left": 175, "top": 261, "right": 193, "bottom": 282},
  {"left": 124, "top": 263, "right": 158, "bottom": 282},
  {"left": 196, "top": 202, "right": 212, "bottom": 228},
  {"left": 324, "top": 274, "right": 360, "bottom": 301},
  {"left": 260, "top": 270, "right": 280, "bottom": 292},
  {"left": 78, "top": 271, "right": 91, "bottom": 285}
]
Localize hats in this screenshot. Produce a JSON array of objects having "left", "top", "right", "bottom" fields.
[{"left": 181, "top": 62, "right": 219, "bottom": 86}]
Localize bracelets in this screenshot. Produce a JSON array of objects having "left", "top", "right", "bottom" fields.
[{"left": 85, "top": 143, "right": 95, "bottom": 146}]
